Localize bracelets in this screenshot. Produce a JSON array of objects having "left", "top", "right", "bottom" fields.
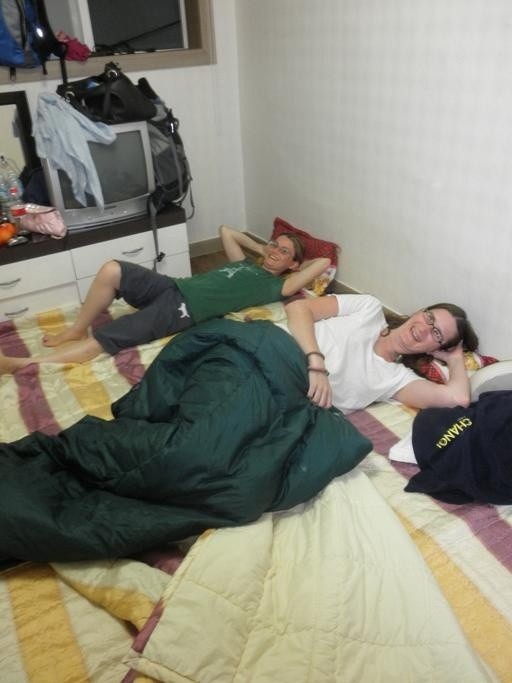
[
  {"left": 307, "top": 352, "right": 325, "bottom": 360},
  {"left": 307, "top": 366, "right": 330, "bottom": 376}
]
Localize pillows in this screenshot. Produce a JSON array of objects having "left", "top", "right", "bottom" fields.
[
  {"left": 255, "top": 217, "right": 340, "bottom": 297},
  {"left": 384, "top": 313, "right": 498, "bottom": 415}
]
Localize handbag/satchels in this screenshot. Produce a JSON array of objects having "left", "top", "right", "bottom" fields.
[
  {"left": 9, "top": 202, "right": 67, "bottom": 238},
  {"left": 56, "top": 62, "right": 158, "bottom": 124}
]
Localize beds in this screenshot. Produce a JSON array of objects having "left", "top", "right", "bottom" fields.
[{"left": 0, "top": 278, "right": 512, "bottom": 683}]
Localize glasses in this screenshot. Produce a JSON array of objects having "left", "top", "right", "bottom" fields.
[
  {"left": 267, "top": 239, "right": 295, "bottom": 258},
  {"left": 423, "top": 308, "right": 446, "bottom": 349}
]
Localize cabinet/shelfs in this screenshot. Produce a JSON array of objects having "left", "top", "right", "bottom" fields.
[{"left": 0, "top": 224, "right": 192, "bottom": 323}]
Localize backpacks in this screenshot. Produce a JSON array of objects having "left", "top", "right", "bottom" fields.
[
  {"left": 135, "top": 72, "right": 191, "bottom": 214},
  {"left": 0, "top": 0, "right": 68, "bottom": 82}
]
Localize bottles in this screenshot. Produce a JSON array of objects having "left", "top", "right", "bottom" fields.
[{"left": 0, "top": 155, "right": 26, "bottom": 240}]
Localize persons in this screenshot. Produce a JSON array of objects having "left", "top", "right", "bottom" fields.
[
  {"left": 273, "top": 294, "right": 478, "bottom": 415},
  {"left": 0, "top": 225, "right": 332, "bottom": 376}
]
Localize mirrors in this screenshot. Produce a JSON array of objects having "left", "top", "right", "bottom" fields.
[{"left": 0, "top": 90, "right": 42, "bottom": 207}]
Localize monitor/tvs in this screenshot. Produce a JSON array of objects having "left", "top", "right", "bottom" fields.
[{"left": 43, "top": 120, "right": 157, "bottom": 236}]
